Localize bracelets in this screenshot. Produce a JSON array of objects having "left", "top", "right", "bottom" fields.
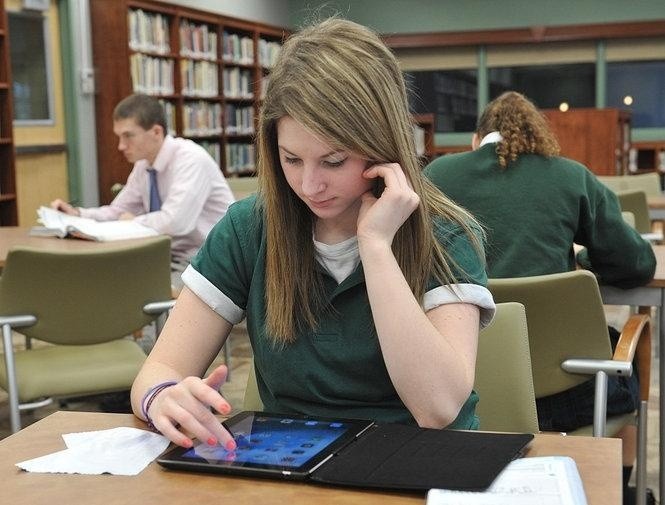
[{"left": 140, "top": 380, "right": 178, "bottom": 436}]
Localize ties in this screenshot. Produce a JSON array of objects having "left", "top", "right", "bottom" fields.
[{"left": 147, "top": 168, "right": 161, "bottom": 211}]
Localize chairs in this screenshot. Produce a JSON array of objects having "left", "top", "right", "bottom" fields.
[
  {"left": 595, "top": 172, "right": 662, "bottom": 195},
  {"left": 243, "top": 302, "right": 542, "bottom": 435},
  {"left": 0, "top": 235, "right": 177, "bottom": 440},
  {"left": 485, "top": 268, "right": 653, "bottom": 505},
  {"left": 617, "top": 190, "right": 664, "bottom": 244}
]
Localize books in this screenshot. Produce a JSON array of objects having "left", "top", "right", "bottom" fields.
[
  {"left": 425, "top": 455, "right": 587, "bottom": 505},
  {"left": 28, "top": 203, "right": 160, "bottom": 244},
  {"left": 125, "top": 4, "right": 282, "bottom": 176}
]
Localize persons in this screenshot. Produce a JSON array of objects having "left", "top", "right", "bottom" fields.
[
  {"left": 43, "top": 89, "right": 238, "bottom": 359},
  {"left": 127, "top": 14, "right": 499, "bottom": 458},
  {"left": 421, "top": 88, "right": 661, "bottom": 505}
]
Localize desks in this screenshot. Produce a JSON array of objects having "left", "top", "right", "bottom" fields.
[
  {"left": 598, "top": 245, "right": 665, "bottom": 505},
  {"left": 0, "top": 410, "right": 623, "bottom": 505},
  {"left": 646, "top": 195, "right": 665, "bottom": 210},
  {"left": 0, "top": 227, "right": 154, "bottom": 341}
]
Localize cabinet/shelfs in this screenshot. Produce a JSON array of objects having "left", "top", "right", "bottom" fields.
[
  {"left": 539, "top": 107, "right": 632, "bottom": 176},
  {"left": 0, "top": 1, "right": 19, "bottom": 229},
  {"left": 627, "top": 141, "right": 665, "bottom": 187},
  {"left": 88, "top": 0, "right": 295, "bottom": 207},
  {"left": 408, "top": 112, "right": 436, "bottom": 171}
]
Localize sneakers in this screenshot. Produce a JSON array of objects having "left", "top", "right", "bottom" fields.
[{"left": 624, "top": 484, "right": 654, "bottom": 505}]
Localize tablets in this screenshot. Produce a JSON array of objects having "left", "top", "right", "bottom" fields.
[{"left": 157, "top": 411, "right": 375, "bottom": 482}]
